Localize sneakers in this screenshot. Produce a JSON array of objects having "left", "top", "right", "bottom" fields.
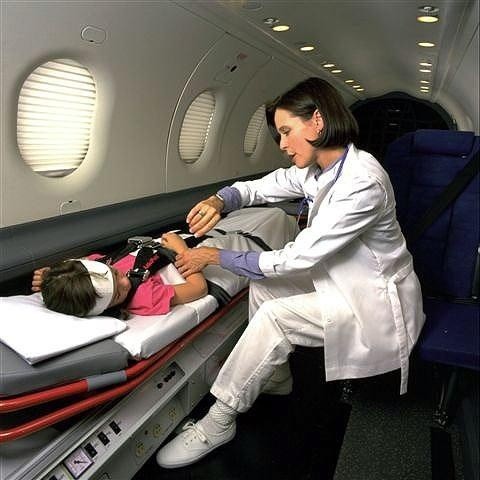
[
  {"left": 156, "top": 419, "right": 237, "bottom": 469},
  {"left": 261, "top": 374, "right": 293, "bottom": 396}
]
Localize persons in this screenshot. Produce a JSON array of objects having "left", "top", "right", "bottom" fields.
[
  {"left": 32, "top": 208, "right": 287, "bottom": 318},
  {"left": 155, "top": 76, "right": 428, "bottom": 469}
]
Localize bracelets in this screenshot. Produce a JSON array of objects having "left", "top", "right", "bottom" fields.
[{"left": 214, "top": 193, "right": 225, "bottom": 202}]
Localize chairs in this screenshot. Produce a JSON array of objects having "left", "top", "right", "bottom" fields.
[{"left": 377, "top": 129, "right": 480, "bottom": 426}]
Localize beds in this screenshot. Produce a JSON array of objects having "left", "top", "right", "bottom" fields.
[{"left": 0, "top": 214, "right": 297, "bottom": 441}]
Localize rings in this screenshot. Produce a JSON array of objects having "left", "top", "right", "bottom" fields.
[{"left": 198, "top": 211, "right": 205, "bottom": 217}]
